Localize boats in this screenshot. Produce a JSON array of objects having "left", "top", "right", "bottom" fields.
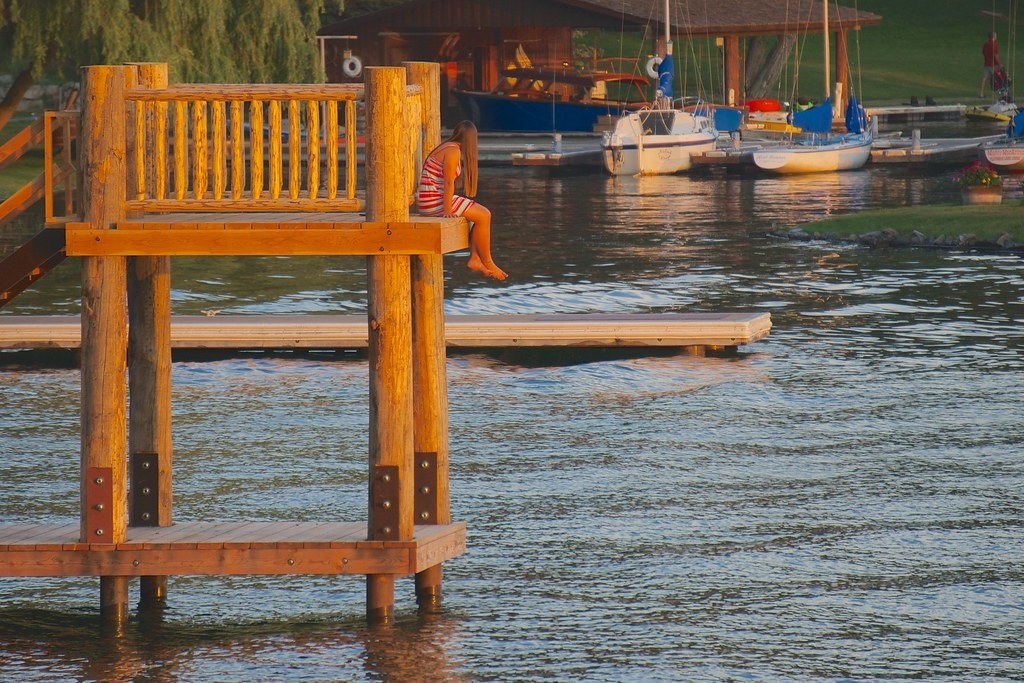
[
  {"left": 977, "top": 139, "right": 1024, "bottom": 171},
  {"left": 449, "top": 66, "right": 655, "bottom": 136}
]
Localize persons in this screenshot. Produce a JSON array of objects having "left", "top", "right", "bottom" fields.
[
  {"left": 979, "top": 31, "right": 1003, "bottom": 98},
  {"left": 419, "top": 121, "right": 508, "bottom": 280}
]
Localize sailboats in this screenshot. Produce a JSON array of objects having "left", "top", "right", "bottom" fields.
[
  {"left": 598, "top": 0, "right": 719, "bottom": 175},
  {"left": 751, "top": 0, "right": 875, "bottom": 173}
]
